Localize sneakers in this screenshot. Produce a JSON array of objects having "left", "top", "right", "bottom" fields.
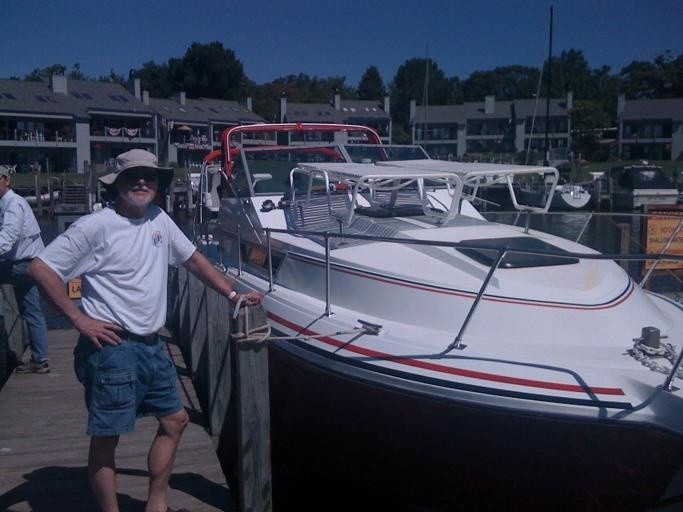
[{"left": 15, "top": 355, "right": 51, "bottom": 374}]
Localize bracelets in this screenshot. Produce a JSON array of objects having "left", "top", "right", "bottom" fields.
[{"left": 228, "top": 290, "right": 236, "bottom": 302}]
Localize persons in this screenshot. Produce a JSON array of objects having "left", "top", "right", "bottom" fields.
[
  {"left": 89, "top": 121, "right": 105, "bottom": 137},
  {"left": 0, "top": 166, "right": 50, "bottom": 375},
  {"left": 26, "top": 149, "right": 267, "bottom": 511},
  {"left": 428, "top": 144, "right": 537, "bottom": 165},
  {"left": 172, "top": 175, "right": 210, "bottom": 248},
  {"left": 0, "top": 119, "right": 74, "bottom": 143}
]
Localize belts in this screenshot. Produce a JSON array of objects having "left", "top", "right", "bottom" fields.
[{"left": 118, "top": 329, "right": 160, "bottom": 345}]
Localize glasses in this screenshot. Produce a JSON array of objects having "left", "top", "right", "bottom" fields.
[{"left": 121, "top": 168, "right": 159, "bottom": 182}]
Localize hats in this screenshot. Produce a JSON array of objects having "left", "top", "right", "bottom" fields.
[
  {"left": 96, "top": 149, "right": 175, "bottom": 198},
  {"left": 0, "top": 165, "right": 10, "bottom": 179}
]
